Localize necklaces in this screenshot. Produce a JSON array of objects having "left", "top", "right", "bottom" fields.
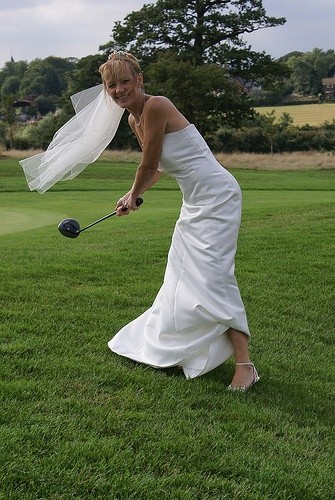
[{"left": 135, "top": 113, "right": 143, "bottom": 126}]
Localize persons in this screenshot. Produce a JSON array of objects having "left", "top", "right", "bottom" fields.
[{"left": 98, "top": 50, "right": 263, "bottom": 392}]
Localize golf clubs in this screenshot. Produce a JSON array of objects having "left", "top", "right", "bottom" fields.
[{"left": 57, "top": 198, "right": 143, "bottom": 238}]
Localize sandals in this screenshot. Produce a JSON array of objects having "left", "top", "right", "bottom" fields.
[{"left": 226, "top": 361, "right": 261, "bottom": 390}]
